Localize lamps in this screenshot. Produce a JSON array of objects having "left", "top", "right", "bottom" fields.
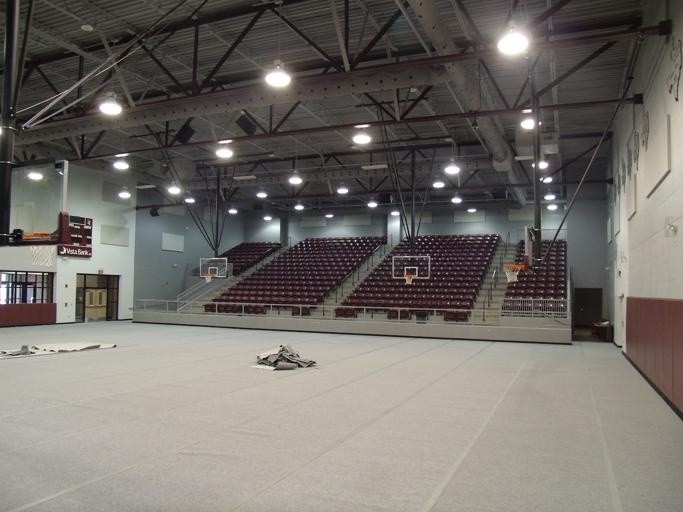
[
  {"left": 496, "top": 0, "right": 530, "bottom": 58},
  {"left": 262, "top": 1, "right": 290, "bottom": 89},
  {"left": 97, "top": 36, "right": 124, "bottom": 116}
]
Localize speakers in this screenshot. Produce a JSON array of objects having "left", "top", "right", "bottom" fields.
[
  {"left": 254, "top": 202, "right": 265, "bottom": 209},
  {"left": 149, "top": 207, "right": 160, "bottom": 217},
  {"left": 236, "top": 115, "right": 257, "bottom": 136},
  {"left": 377, "top": 193, "right": 393, "bottom": 204},
  {"left": 178, "top": 126, "right": 195, "bottom": 144},
  {"left": 493, "top": 188, "right": 507, "bottom": 198}
]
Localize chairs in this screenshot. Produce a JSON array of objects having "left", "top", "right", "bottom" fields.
[{"left": 590, "top": 320, "right": 598, "bottom": 336}]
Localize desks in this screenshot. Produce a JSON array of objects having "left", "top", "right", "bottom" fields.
[{"left": 597, "top": 323, "right": 612, "bottom": 341}]
